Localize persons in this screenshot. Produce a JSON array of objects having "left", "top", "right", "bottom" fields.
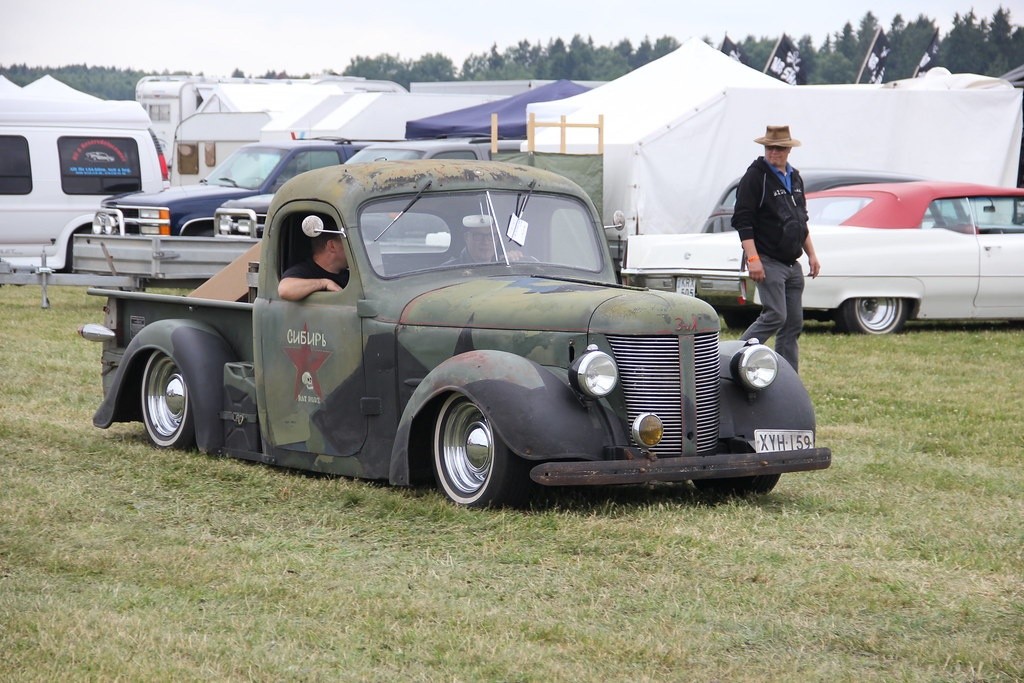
[
  {"left": 278, "top": 215, "right": 351, "bottom": 301},
  {"left": 443, "top": 208, "right": 525, "bottom": 267},
  {"left": 731, "top": 126, "right": 820, "bottom": 374}
]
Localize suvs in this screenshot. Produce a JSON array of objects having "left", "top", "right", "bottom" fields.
[
  {"left": 92, "top": 135, "right": 369, "bottom": 237},
  {"left": 215, "top": 135, "right": 527, "bottom": 241}
]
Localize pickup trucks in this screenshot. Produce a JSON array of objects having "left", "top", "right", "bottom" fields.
[{"left": 75, "top": 157, "right": 832, "bottom": 507}]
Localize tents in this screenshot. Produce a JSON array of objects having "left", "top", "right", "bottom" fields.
[
  {"left": 197, "top": 37, "right": 1022, "bottom": 242},
  {"left": 0, "top": 74, "right": 150, "bottom": 124}
]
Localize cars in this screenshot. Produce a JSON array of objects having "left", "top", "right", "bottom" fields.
[
  {"left": 618, "top": 179, "right": 1024, "bottom": 336},
  {"left": 702, "top": 171, "right": 971, "bottom": 232}
]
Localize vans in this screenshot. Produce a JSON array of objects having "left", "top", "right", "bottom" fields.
[{"left": 0, "top": 100, "right": 170, "bottom": 271}]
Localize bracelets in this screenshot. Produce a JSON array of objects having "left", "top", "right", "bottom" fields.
[{"left": 746, "top": 255, "right": 759, "bottom": 263}]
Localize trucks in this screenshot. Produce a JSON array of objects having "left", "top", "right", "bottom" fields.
[{"left": 71, "top": 233, "right": 263, "bottom": 293}]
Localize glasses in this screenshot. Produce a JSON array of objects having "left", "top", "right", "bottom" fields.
[
  {"left": 766, "top": 145, "right": 791, "bottom": 151},
  {"left": 466, "top": 231, "right": 497, "bottom": 242}
]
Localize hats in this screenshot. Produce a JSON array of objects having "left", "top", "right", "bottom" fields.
[{"left": 755, "top": 125, "right": 800, "bottom": 147}]
